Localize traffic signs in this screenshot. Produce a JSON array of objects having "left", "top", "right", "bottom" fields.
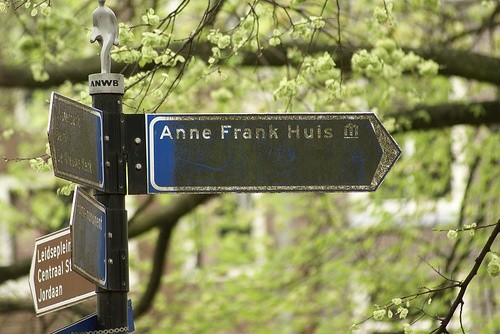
[
  {"left": 144, "top": 112, "right": 404, "bottom": 195},
  {"left": 68, "top": 184, "right": 109, "bottom": 281},
  {"left": 46, "top": 92, "right": 104, "bottom": 193},
  {"left": 28, "top": 226, "right": 98, "bottom": 318},
  {"left": 50, "top": 299, "right": 135, "bottom": 334}
]
